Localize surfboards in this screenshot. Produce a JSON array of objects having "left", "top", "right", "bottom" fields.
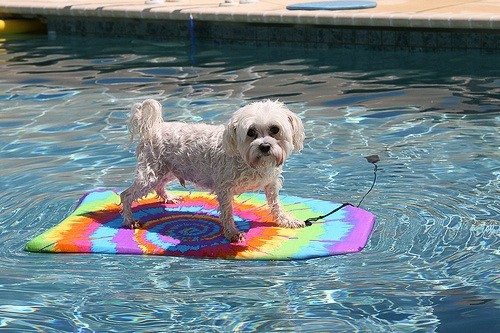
[{"left": 21, "top": 183, "right": 375, "bottom": 260}]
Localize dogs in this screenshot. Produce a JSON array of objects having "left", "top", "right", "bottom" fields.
[{"left": 114, "top": 98, "right": 306, "bottom": 243}]
